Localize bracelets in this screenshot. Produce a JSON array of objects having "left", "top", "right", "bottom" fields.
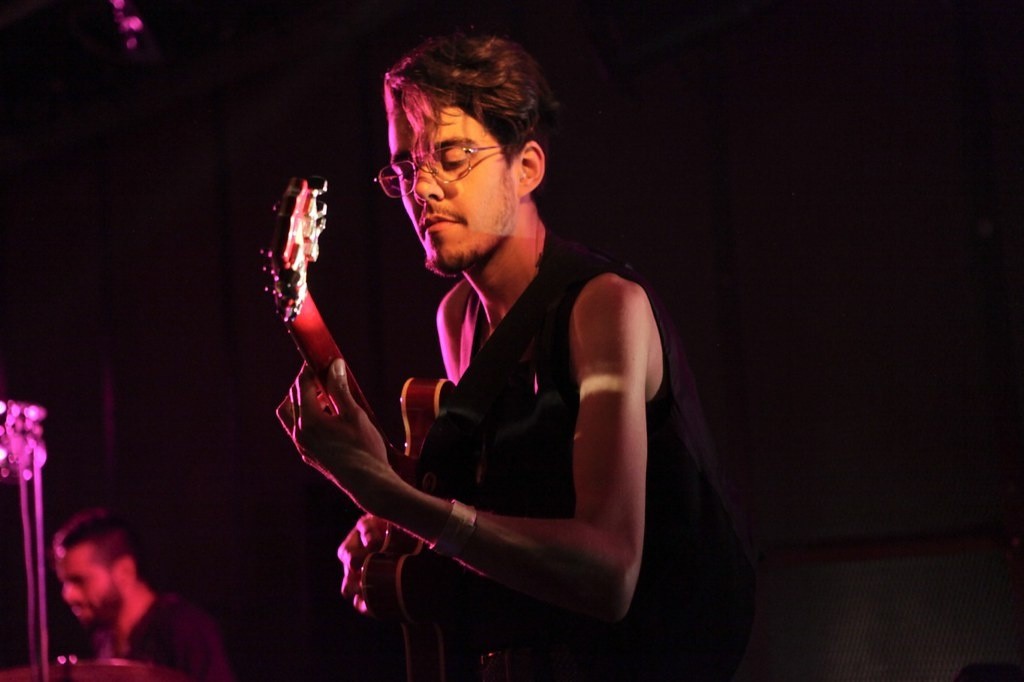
[{"left": 428, "top": 499, "right": 476, "bottom": 559}]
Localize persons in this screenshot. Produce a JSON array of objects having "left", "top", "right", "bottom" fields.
[
  {"left": 51, "top": 509, "right": 234, "bottom": 682},
  {"left": 277, "top": 36, "right": 767, "bottom": 682}
]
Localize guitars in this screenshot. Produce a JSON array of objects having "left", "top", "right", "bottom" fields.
[{"left": 256, "top": 171, "right": 486, "bottom": 682}]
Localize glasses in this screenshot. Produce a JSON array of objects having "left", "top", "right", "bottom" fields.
[{"left": 374, "top": 145, "right": 505, "bottom": 198}]
